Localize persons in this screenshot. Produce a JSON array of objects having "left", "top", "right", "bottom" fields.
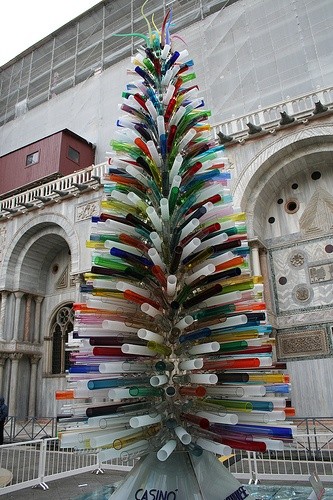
[{"left": 0, "top": 397, "right": 8, "bottom": 445}]
